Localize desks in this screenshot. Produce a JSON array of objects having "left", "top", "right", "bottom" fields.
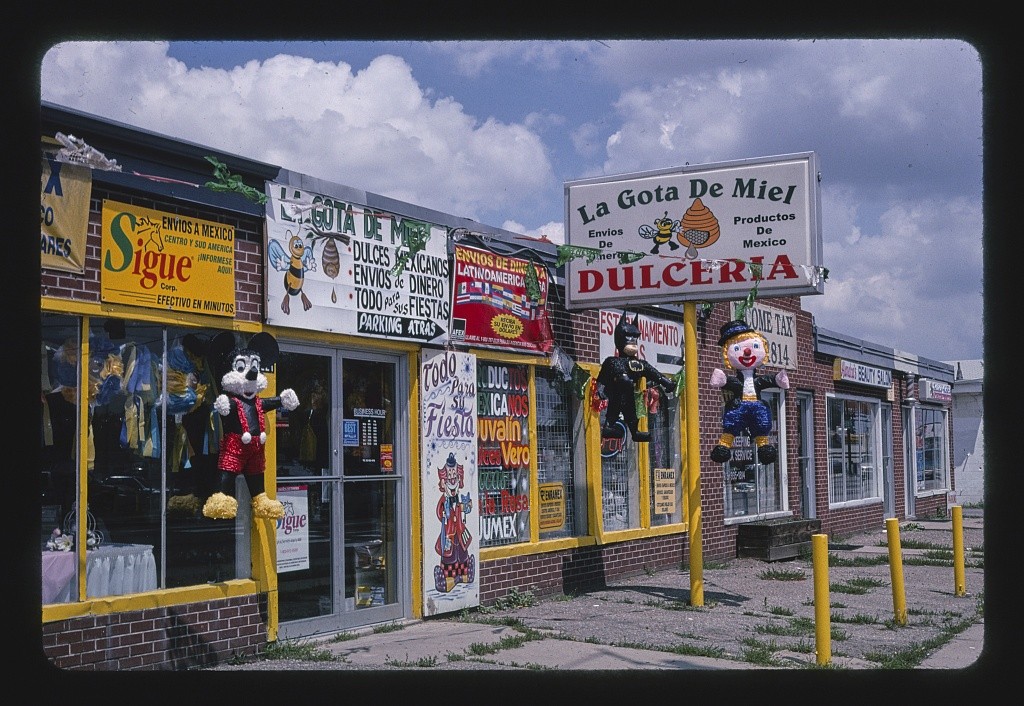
[{"left": 41, "top": 542, "right": 157, "bottom": 608}]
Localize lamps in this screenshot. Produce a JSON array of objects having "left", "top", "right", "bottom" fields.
[{"left": 902, "top": 371, "right": 916, "bottom": 409}]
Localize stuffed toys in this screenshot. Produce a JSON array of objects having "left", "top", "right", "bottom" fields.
[
  {"left": 202, "top": 332, "right": 300, "bottom": 519},
  {"left": 710, "top": 320, "right": 789, "bottom": 466},
  {"left": 596, "top": 306, "right": 676, "bottom": 441}
]
